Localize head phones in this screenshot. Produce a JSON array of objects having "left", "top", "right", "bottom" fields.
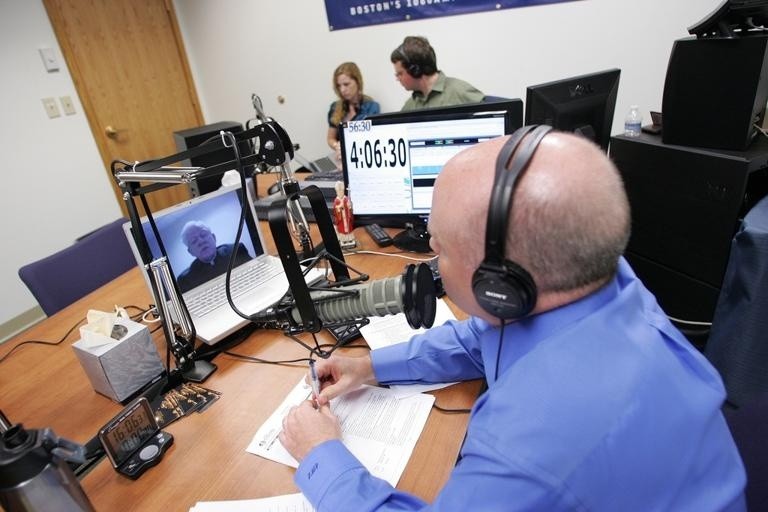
[
  {"left": 397, "top": 45, "right": 422, "bottom": 78},
  {"left": 470, "top": 124, "right": 552, "bottom": 318}
]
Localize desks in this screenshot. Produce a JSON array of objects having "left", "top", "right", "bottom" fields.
[
  {"left": 608, "top": 131, "right": 768, "bottom": 350},
  {"left": 0, "top": 174, "right": 484, "bottom": 511}
]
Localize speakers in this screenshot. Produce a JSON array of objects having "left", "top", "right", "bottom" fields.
[
  {"left": 608, "top": 130, "right": 768, "bottom": 288},
  {"left": 622, "top": 249, "right": 723, "bottom": 355},
  {"left": 661, "top": 32, "right": 768, "bottom": 152},
  {"left": 172, "top": 121, "right": 254, "bottom": 197}
]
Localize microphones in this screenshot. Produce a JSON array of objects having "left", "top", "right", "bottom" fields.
[{"left": 250, "top": 262, "right": 436, "bottom": 329}]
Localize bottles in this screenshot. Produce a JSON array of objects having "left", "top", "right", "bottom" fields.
[
  {"left": 624, "top": 104, "right": 642, "bottom": 138},
  {"left": 0, "top": 410, "right": 99, "bottom": 512}
]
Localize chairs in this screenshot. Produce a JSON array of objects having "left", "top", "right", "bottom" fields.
[
  {"left": 701, "top": 197, "right": 768, "bottom": 512},
  {"left": 17, "top": 216, "right": 138, "bottom": 316}
]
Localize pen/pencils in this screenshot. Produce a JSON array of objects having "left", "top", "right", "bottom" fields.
[{"left": 309, "top": 361, "right": 320, "bottom": 409}]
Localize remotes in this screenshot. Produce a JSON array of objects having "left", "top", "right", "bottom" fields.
[{"left": 364, "top": 223, "right": 393, "bottom": 247}]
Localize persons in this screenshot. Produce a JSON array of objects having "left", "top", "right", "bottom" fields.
[
  {"left": 279, "top": 128, "right": 748, "bottom": 512},
  {"left": 390, "top": 36, "right": 485, "bottom": 115},
  {"left": 176, "top": 219, "right": 254, "bottom": 293},
  {"left": 327, "top": 62, "right": 381, "bottom": 166}
]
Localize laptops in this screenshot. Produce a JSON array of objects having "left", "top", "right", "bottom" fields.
[{"left": 121, "top": 181, "right": 325, "bottom": 346}]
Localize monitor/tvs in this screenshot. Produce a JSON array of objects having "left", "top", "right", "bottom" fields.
[
  {"left": 524, "top": 68, "right": 622, "bottom": 155},
  {"left": 337, "top": 98, "right": 524, "bottom": 251}
]
